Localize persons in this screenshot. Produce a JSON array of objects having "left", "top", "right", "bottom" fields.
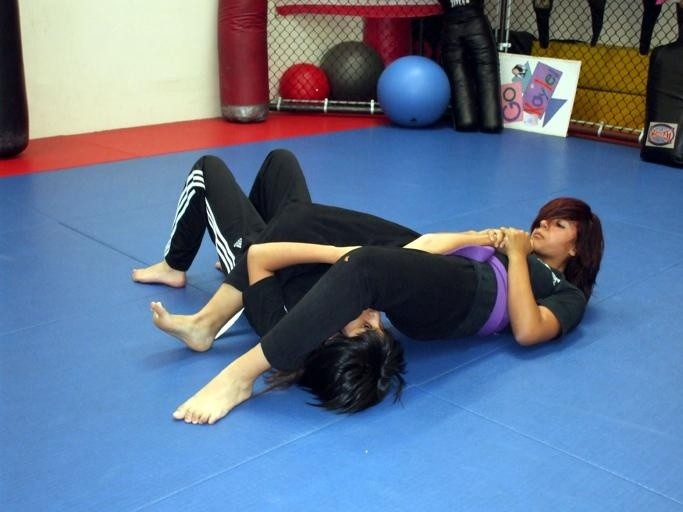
[
  {"left": 146, "top": 195, "right": 604, "bottom": 424},
  {"left": 129, "top": 147, "right": 409, "bottom": 418}
]
